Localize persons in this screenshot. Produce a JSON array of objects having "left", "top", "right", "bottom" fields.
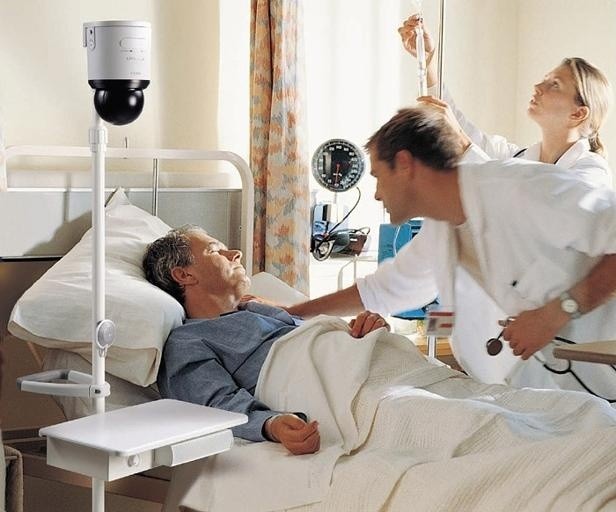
[
  {"left": 398, "top": 13, "right": 611, "bottom": 184},
  {"left": 144, "top": 226, "right": 390, "bottom": 455},
  {"left": 240, "top": 109, "right": 616, "bottom": 410}
]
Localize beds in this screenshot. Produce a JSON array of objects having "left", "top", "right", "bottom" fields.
[{"left": 0, "top": 145, "right": 616, "bottom": 512}]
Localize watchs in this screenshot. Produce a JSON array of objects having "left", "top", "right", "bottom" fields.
[{"left": 559, "top": 290, "right": 582, "bottom": 319}]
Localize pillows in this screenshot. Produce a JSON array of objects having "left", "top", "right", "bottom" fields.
[{"left": 6, "top": 184, "right": 186, "bottom": 388}]
